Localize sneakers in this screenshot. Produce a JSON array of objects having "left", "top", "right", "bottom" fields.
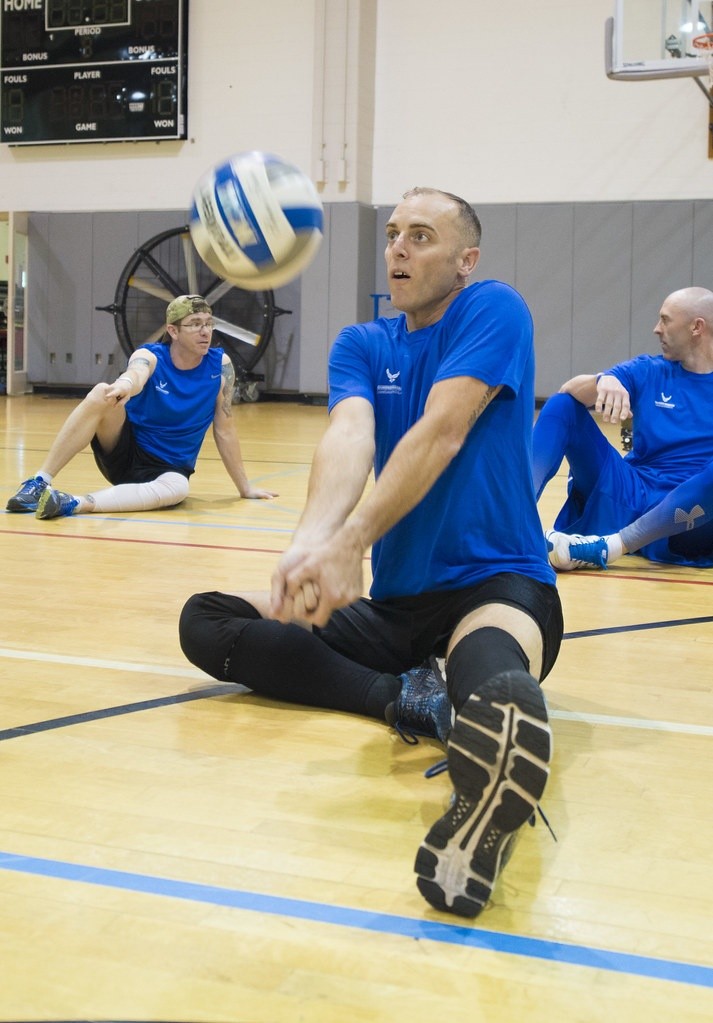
[
  {"left": 35, "top": 484, "right": 79, "bottom": 519},
  {"left": 546, "top": 529, "right": 610, "bottom": 572},
  {"left": 386, "top": 656, "right": 454, "bottom": 746},
  {"left": 6, "top": 476, "right": 53, "bottom": 512},
  {"left": 414, "top": 670, "right": 558, "bottom": 916}
]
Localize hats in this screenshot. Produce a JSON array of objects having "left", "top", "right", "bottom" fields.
[{"left": 165, "top": 295, "right": 212, "bottom": 326}]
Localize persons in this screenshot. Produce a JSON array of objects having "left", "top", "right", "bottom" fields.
[
  {"left": 179, "top": 187, "right": 565, "bottom": 922},
  {"left": 532, "top": 286, "right": 713, "bottom": 572},
  {"left": 6, "top": 297, "right": 278, "bottom": 520}
]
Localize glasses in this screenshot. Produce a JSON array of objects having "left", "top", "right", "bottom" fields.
[{"left": 172, "top": 322, "right": 215, "bottom": 331}]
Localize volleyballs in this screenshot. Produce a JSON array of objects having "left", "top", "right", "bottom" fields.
[{"left": 188, "top": 151, "right": 326, "bottom": 290}]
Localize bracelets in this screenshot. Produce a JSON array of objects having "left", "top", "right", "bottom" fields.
[
  {"left": 596, "top": 374, "right": 601, "bottom": 382},
  {"left": 119, "top": 378, "right": 134, "bottom": 385}
]
